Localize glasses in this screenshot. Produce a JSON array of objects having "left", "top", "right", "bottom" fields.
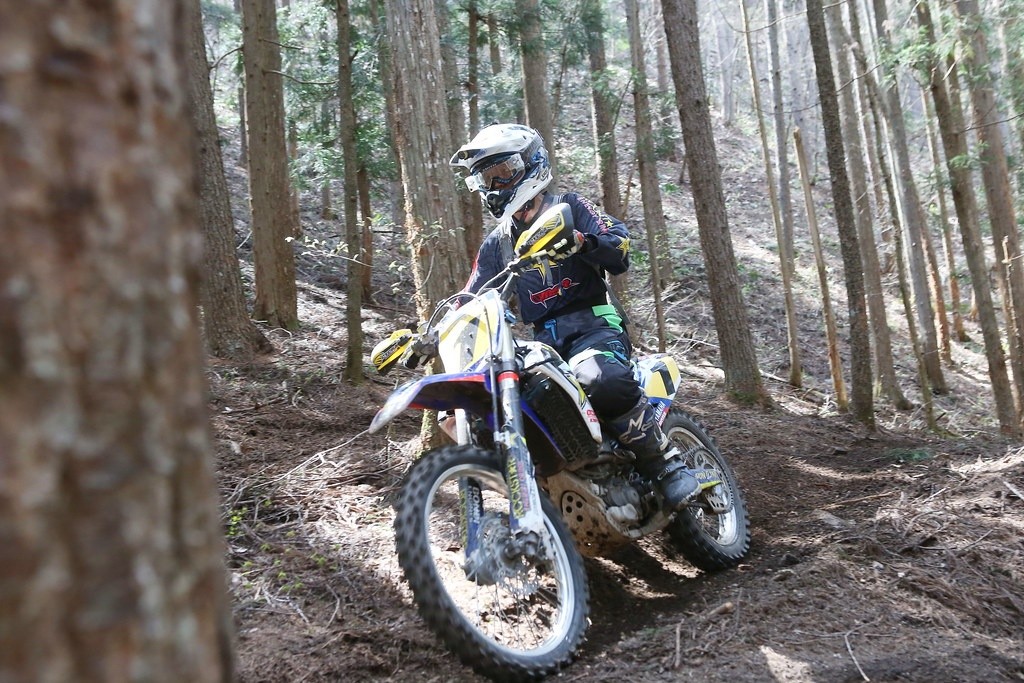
[{"left": 463, "top": 128, "right": 545, "bottom": 193}]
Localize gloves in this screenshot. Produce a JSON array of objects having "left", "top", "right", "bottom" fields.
[
  {"left": 547, "top": 229, "right": 586, "bottom": 263},
  {"left": 403, "top": 331, "right": 439, "bottom": 371}
]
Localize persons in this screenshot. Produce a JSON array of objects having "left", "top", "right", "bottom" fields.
[{"left": 402, "top": 124, "right": 703, "bottom": 519}]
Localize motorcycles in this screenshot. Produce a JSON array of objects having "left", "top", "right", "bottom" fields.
[{"left": 365, "top": 202, "right": 753, "bottom": 683}]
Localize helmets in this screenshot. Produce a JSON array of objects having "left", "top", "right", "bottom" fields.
[{"left": 448, "top": 123, "right": 553, "bottom": 224}]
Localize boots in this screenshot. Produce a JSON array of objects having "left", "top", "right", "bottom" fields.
[{"left": 598, "top": 387, "right": 702, "bottom": 512}]
